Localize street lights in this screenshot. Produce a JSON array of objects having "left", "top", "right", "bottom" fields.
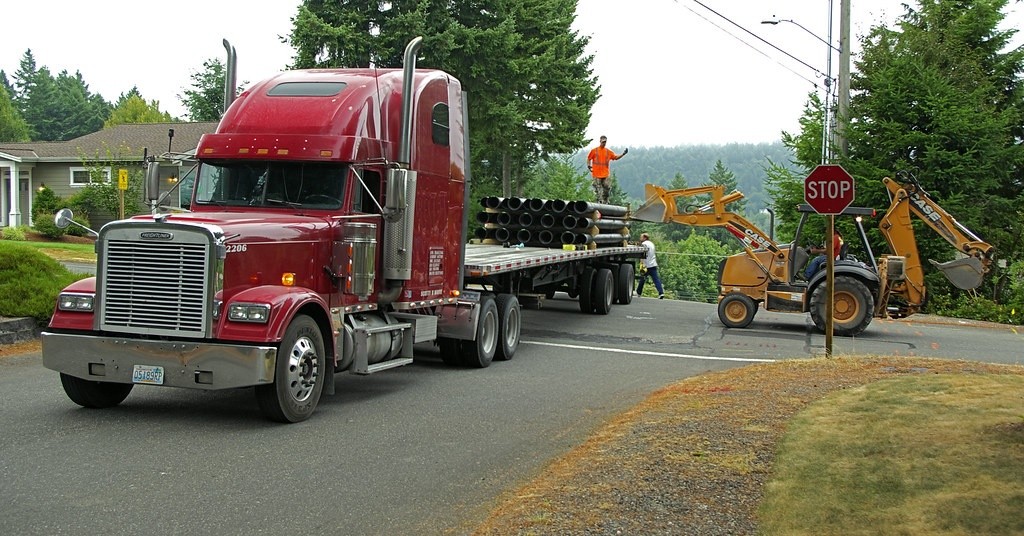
[{"left": 761, "top": 19, "right": 850, "bottom": 160}]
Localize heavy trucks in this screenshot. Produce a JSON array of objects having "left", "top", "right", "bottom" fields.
[{"left": 40, "top": 34, "right": 649, "bottom": 424}]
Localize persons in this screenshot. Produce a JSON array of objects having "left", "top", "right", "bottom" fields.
[
  {"left": 586, "top": 135, "right": 628, "bottom": 205},
  {"left": 632, "top": 233, "right": 665, "bottom": 299},
  {"left": 796, "top": 224, "right": 840, "bottom": 282}
]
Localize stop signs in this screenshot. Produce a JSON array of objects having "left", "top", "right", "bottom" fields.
[{"left": 804, "top": 164, "right": 855, "bottom": 216}]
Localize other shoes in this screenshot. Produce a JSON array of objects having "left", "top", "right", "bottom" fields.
[
  {"left": 796, "top": 271, "right": 808, "bottom": 282},
  {"left": 658, "top": 293, "right": 664, "bottom": 299},
  {"left": 633, "top": 290, "right": 640, "bottom": 297}
]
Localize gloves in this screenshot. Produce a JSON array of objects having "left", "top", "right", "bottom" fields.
[
  {"left": 622, "top": 148, "right": 629, "bottom": 155},
  {"left": 587, "top": 165, "right": 592, "bottom": 171}
]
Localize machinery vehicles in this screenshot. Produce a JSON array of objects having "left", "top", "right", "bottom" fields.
[{"left": 626, "top": 168, "right": 999, "bottom": 338}]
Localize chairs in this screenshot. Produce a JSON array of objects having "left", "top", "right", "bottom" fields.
[{"left": 818, "top": 243, "right": 848, "bottom": 270}]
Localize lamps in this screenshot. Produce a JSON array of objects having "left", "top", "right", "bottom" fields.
[{"left": 172, "top": 173, "right": 178, "bottom": 183}]
